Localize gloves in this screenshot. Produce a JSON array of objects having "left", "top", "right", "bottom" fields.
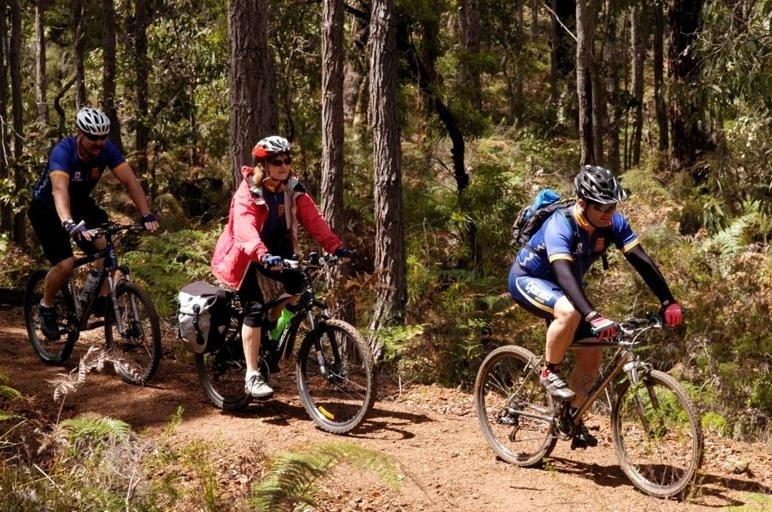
[
  {"left": 658, "top": 299, "right": 683, "bottom": 327},
  {"left": 586, "top": 311, "right": 617, "bottom": 340}
]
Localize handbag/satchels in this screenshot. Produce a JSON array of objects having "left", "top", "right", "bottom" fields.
[{"left": 175, "top": 281, "right": 230, "bottom": 355}]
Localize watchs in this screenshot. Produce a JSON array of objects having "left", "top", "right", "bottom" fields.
[{"left": 61, "top": 218, "right": 74, "bottom": 228}]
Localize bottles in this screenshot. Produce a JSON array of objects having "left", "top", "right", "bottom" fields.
[
  {"left": 79, "top": 267, "right": 98, "bottom": 302},
  {"left": 270, "top": 303, "right": 296, "bottom": 341}
]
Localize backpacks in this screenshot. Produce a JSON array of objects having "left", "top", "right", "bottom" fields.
[{"left": 512, "top": 188, "right": 615, "bottom": 269}]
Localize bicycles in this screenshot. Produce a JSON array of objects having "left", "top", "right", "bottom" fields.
[
  {"left": 474, "top": 308, "right": 702, "bottom": 499},
  {"left": 178, "top": 248, "right": 376, "bottom": 437},
  {"left": 24, "top": 217, "right": 163, "bottom": 386}
]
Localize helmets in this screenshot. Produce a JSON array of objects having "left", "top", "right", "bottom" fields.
[
  {"left": 251, "top": 136, "right": 291, "bottom": 160},
  {"left": 575, "top": 164, "right": 628, "bottom": 205},
  {"left": 75, "top": 107, "right": 111, "bottom": 135}
]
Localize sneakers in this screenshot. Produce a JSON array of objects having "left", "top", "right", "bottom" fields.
[
  {"left": 540, "top": 369, "right": 576, "bottom": 402},
  {"left": 560, "top": 411, "right": 597, "bottom": 446},
  {"left": 36, "top": 300, "right": 61, "bottom": 341},
  {"left": 244, "top": 370, "right": 273, "bottom": 398}
]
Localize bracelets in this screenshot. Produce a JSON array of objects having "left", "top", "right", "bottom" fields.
[{"left": 585, "top": 311, "right": 598, "bottom": 322}]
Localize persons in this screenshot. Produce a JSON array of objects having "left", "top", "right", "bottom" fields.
[
  {"left": 507, "top": 163, "right": 683, "bottom": 447},
  {"left": 27, "top": 105, "right": 160, "bottom": 339},
  {"left": 211, "top": 135, "right": 357, "bottom": 398}
]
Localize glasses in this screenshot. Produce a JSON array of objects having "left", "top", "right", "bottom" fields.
[
  {"left": 82, "top": 132, "right": 109, "bottom": 141},
  {"left": 270, "top": 159, "right": 294, "bottom": 166},
  {"left": 593, "top": 202, "right": 616, "bottom": 213}
]
